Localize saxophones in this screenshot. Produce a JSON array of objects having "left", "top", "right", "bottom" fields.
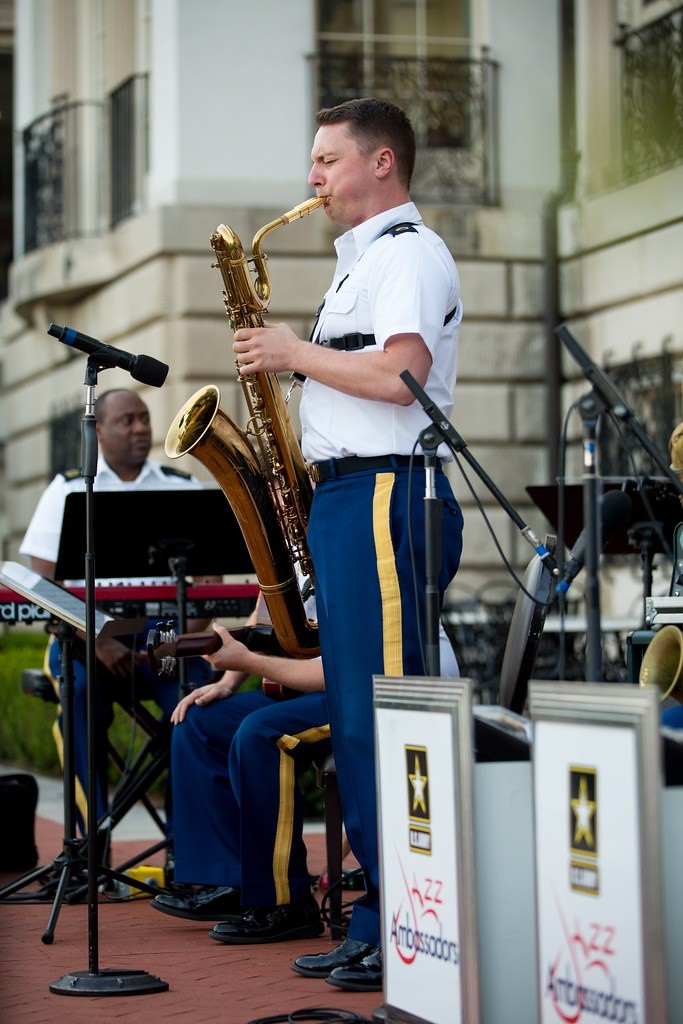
[
  {"left": 164, "top": 196, "right": 329, "bottom": 660},
  {"left": 637, "top": 418, "right": 683, "bottom": 701}
]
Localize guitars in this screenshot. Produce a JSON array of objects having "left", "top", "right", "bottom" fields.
[{"left": 146, "top": 620, "right": 323, "bottom": 703}]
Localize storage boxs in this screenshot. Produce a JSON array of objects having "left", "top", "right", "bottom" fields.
[{"left": 646, "top": 597, "right": 683, "bottom": 624}]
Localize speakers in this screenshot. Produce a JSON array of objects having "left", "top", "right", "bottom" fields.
[{"left": 0, "top": 772, "right": 39, "bottom": 873}]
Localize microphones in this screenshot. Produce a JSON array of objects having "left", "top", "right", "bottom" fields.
[
  {"left": 548, "top": 491, "right": 632, "bottom": 609},
  {"left": 46, "top": 323, "right": 169, "bottom": 388}
]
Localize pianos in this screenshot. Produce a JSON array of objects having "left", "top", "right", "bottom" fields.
[{"left": 0, "top": 579, "right": 260, "bottom": 623}]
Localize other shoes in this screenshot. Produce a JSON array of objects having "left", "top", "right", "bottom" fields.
[
  {"left": 163, "top": 844, "right": 177, "bottom": 882},
  {"left": 41, "top": 837, "right": 113, "bottom": 893}
]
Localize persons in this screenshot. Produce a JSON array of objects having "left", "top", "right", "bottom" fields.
[
  {"left": 19, "top": 390, "right": 460, "bottom": 944},
  {"left": 234, "top": 99, "right": 466, "bottom": 992}
]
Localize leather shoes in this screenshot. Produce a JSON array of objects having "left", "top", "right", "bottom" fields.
[
  {"left": 325, "top": 944, "right": 383, "bottom": 992},
  {"left": 291, "top": 938, "right": 375, "bottom": 978},
  {"left": 207, "top": 894, "right": 326, "bottom": 945},
  {"left": 151, "top": 886, "right": 243, "bottom": 920}
]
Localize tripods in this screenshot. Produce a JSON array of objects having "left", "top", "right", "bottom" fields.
[{"left": 0, "top": 562, "right": 176, "bottom": 945}]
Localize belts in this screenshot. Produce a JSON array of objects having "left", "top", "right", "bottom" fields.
[{"left": 306, "top": 453, "right": 442, "bottom": 485}]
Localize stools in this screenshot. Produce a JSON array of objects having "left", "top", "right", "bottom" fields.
[
  {"left": 311, "top": 534, "right": 557, "bottom": 942},
  {"left": 22, "top": 666, "right": 225, "bottom": 838}
]
